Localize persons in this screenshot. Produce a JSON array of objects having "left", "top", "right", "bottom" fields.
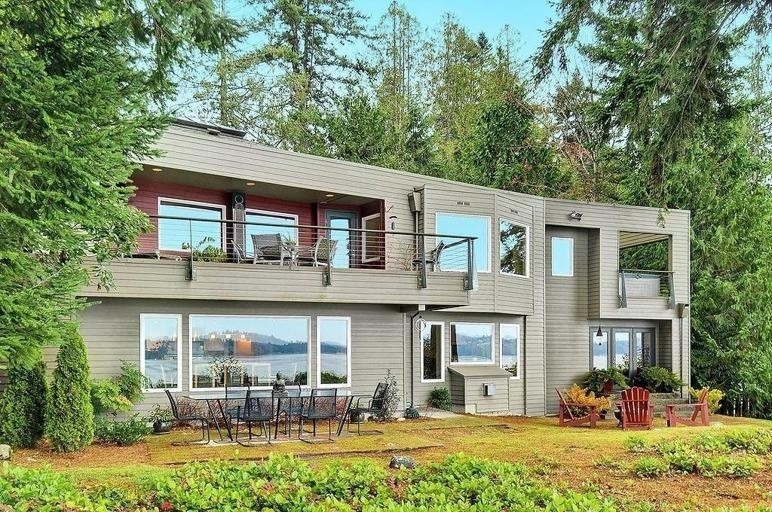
[{"left": 271, "top": 372, "right": 289, "bottom": 395}]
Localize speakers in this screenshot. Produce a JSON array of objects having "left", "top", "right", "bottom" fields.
[{"left": 232, "top": 191, "right": 246, "bottom": 209}]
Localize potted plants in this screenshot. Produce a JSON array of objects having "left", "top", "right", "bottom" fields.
[{"left": 584, "top": 366, "right": 628, "bottom": 395}]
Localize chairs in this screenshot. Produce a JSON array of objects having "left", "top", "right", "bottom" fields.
[
  {"left": 554, "top": 386, "right": 596, "bottom": 429},
  {"left": 164, "top": 382, "right": 390, "bottom": 447},
  {"left": 412, "top": 240, "right": 445, "bottom": 273},
  {"left": 615, "top": 388, "right": 654, "bottom": 432},
  {"left": 665, "top": 388, "right": 710, "bottom": 426},
  {"left": 233, "top": 234, "right": 338, "bottom": 267}
]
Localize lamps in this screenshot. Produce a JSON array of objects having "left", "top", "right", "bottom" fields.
[
  {"left": 415, "top": 312, "right": 426, "bottom": 331},
  {"left": 594, "top": 320, "right": 604, "bottom": 347}
]
